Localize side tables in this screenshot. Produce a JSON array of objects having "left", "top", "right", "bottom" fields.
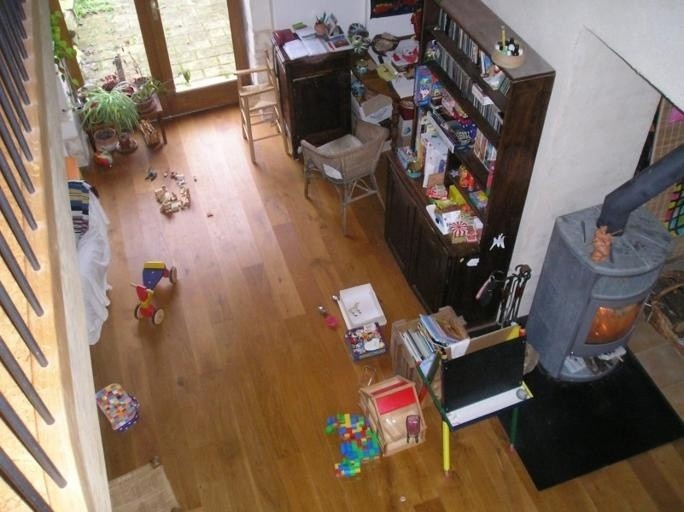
[{"left": 392, "top": 305, "right": 534, "bottom": 477}]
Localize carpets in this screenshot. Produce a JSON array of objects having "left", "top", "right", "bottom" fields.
[{"left": 468, "top": 315, "right": 684, "bottom": 491}]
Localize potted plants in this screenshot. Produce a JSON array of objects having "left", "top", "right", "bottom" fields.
[
  {"left": 74, "top": 71, "right": 167, "bottom": 154},
  {"left": 313, "top": 12, "right": 330, "bottom": 37}
]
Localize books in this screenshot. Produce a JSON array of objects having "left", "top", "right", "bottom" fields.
[
  {"left": 346, "top": 322, "right": 387, "bottom": 361},
  {"left": 413, "top": 7, "right": 512, "bottom": 189},
  {"left": 402, "top": 313, "right": 462, "bottom": 366},
  {"left": 273, "top": 21, "right": 353, "bottom": 62}
]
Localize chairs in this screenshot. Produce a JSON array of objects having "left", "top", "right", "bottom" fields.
[
  {"left": 232, "top": 67, "right": 289, "bottom": 162},
  {"left": 299, "top": 120, "right": 392, "bottom": 235}
]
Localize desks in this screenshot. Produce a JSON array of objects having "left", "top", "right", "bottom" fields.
[{"left": 349, "top": 35, "right": 414, "bottom": 146}]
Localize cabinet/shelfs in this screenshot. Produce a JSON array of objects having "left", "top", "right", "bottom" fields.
[
  {"left": 384, "top": 4, "right": 555, "bottom": 256},
  {"left": 380, "top": 150, "right": 456, "bottom": 318},
  {"left": 271, "top": 32, "right": 353, "bottom": 159}
]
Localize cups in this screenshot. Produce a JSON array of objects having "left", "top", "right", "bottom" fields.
[{"left": 382, "top": 417, "right": 401, "bottom": 439}]
